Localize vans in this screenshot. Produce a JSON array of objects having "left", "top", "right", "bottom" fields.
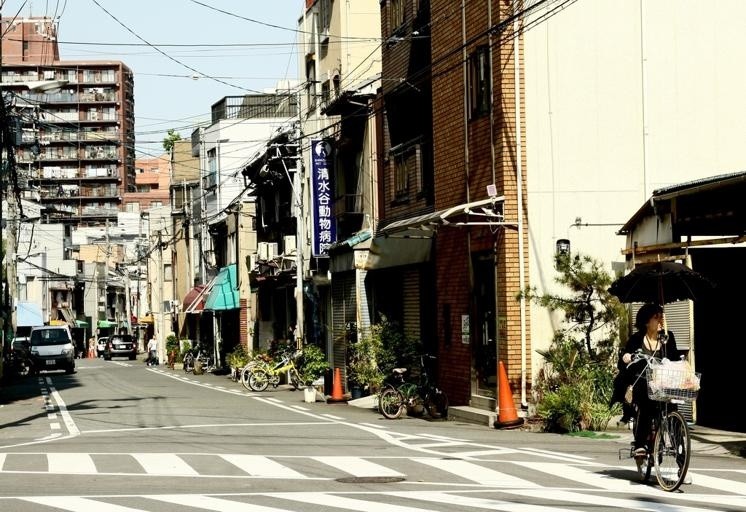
[{"left": 27, "top": 325, "right": 75, "bottom": 375}]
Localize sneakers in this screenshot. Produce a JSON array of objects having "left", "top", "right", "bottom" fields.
[
  {"left": 633, "top": 447, "right": 646, "bottom": 459},
  {"left": 677, "top": 469, "right": 692, "bottom": 484}
]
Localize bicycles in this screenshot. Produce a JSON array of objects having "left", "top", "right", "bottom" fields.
[
  {"left": 182, "top": 344, "right": 212, "bottom": 373},
  {"left": 619, "top": 348, "right": 702, "bottom": 493},
  {"left": 241, "top": 334, "right": 306, "bottom": 392},
  {"left": 379, "top": 353, "right": 449, "bottom": 419}
]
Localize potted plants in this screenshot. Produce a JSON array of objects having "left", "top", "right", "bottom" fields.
[
  {"left": 294, "top": 342, "right": 330, "bottom": 403},
  {"left": 166, "top": 334, "right": 179, "bottom": 368},
  {"left": 514, "top": 245, "right": 625, "bottom": 432},
  {"left": 348, "top": 323, "right": 379, "bottom": 400},
  {"left": 226, "top": 352, "right": 245, "bottom": 379}
]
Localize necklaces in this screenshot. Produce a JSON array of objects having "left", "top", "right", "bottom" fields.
[{"left": 645, "top": 335, "right": 659, "bottom": 356}]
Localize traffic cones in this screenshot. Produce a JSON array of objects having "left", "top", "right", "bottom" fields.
[
  {"left": 494, "top": 360, "right": 524, "bottom": 430},
  {"left": 327, "top": 367, "right": 347, "bottom": 403}
]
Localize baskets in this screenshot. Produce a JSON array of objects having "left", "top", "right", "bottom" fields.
[{"left": 645, "top": 367, "right": 702, "bottom": 404}]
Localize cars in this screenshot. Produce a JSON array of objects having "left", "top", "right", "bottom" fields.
[{"left": 96, "top": 334, "right": 137, "bottom": 360}]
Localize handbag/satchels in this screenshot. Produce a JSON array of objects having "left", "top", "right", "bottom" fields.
[{"left": 624, "top": 385, "right": 633, "bottom": 403}]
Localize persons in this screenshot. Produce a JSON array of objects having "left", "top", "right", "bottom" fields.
[
  {"left": 610, "top": 303, "right": 691, "bottom": 485},
  {"left": 88, "top": 336, "right": 95, "bottom": 359},
  {"left": 147, "top": 335, "right": 157, "bottom": 366}
]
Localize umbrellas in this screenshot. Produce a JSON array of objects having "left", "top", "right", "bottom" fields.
[{"left": 607, "top": 261, "right": 715, "bottom": 342}]
